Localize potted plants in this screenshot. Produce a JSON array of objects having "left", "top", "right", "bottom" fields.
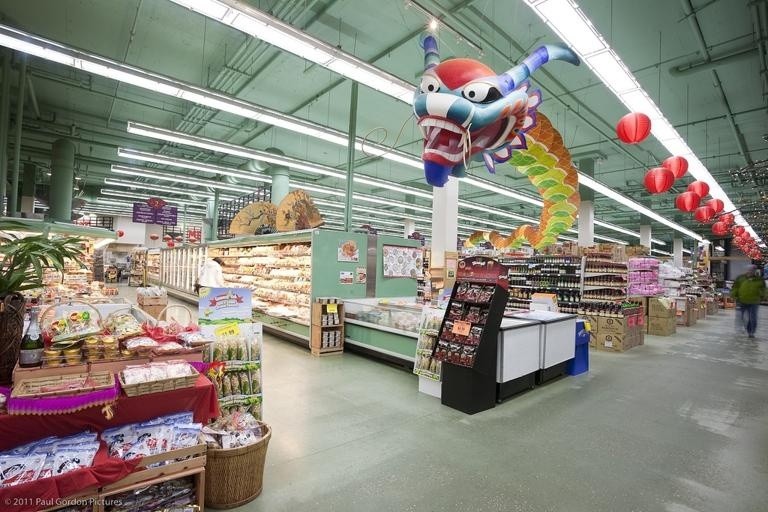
[{"left": 0, "top": 226, "right": 89, "bottom": 384}]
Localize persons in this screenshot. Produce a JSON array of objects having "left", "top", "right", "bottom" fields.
[
  {"left": 197, "top": 257, "right": 225, "bottom": 294},
  {"left": 729, "top": 263, "right": 768, "bottom": 339}
]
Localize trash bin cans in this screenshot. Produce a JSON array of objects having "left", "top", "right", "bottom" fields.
[{"left": 565, "top": 318, "right": 590, "bottom": 376}]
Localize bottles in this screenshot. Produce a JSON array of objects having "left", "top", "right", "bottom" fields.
[
  {"left": 18, "top": 305, "right": 44, "bottom": 368},
  {"left": 508, "top": 256, "right": 624, "bottom": 317}
]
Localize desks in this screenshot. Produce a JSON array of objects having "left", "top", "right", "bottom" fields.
[{"left": 0, "top": 370, "right": 220, "bottom": 449}]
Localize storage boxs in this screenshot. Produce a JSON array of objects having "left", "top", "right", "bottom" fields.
[{"left": 138, "top": 294, "right": 168, "bottom": 306}]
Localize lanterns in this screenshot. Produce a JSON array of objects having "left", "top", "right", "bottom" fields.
[
  {"left": 711, "top": 212, "right": 762, "bottom": 261},
  {"left": 687, "top": 181, "right": 710, "bottom": 198},
  {"left": 150, "top": 232, "right": 198, "bottom": 249},
  {"left": 676, "top": 191, "right": 700, "bottom": 212},
  {"left": 663, "top": 157, "right": 688, "bottom": 180},
  {"left": 706, "top": 198, "right": 724, "bottom": 213},
  {"left": 695, "top": 206, "right": 715, "bottom": 223},
  {"left": 643, "top": 167, "right": 674, "bottom": 194},
  {"left": 617, "top": 111, "right": 651, "bottom": 144}
]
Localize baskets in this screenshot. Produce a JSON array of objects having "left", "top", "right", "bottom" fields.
[
  {"left": 204, "top": 420, "right": 271, "bottom": 509},
  {"left": 11, "top": 371, "right": 117, "bottom": 402},
  {"left": 117, "top": 361, "right": 200, "bottom": 397}
]
